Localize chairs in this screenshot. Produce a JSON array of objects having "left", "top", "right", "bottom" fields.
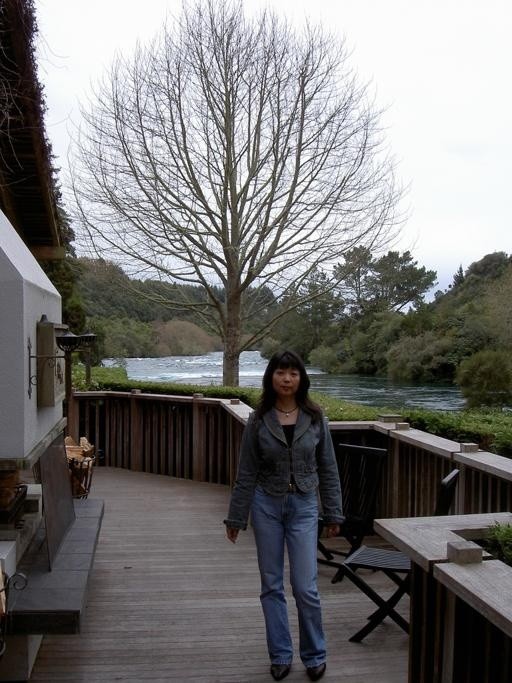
[{"left": 317, "top": 443, "right": 460, "bottom": 642}]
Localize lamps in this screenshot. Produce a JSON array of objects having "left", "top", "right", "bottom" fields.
[{"left": 26, "top": 329, "right": 98, "bottom": 400}]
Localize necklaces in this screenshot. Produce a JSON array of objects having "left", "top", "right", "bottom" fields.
[{"left": 273, "top": 402, "right": 299, "bottom": 416}]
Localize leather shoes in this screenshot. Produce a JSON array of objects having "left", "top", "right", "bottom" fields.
[
  {"left": 271, "top": 664, "right": 291, "bottom": 680},
  {"left": 308, "top": 662, "right": 326, "bottom": 682}
]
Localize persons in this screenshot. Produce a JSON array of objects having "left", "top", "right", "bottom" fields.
[{"left": 222, "top": 346, "right": 348, "bottom": 682}]
